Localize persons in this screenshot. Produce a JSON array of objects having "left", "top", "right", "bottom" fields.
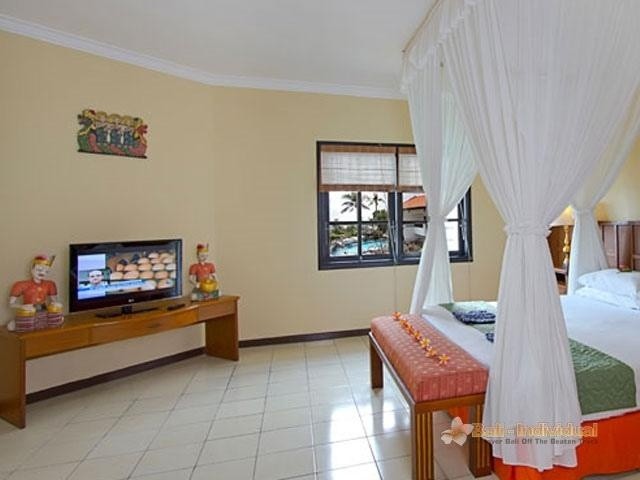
[
  {"left": 189, "top": 241, "right": 221, "bottom": 302},
  {"left": 87, "top": 270, "right": 108, "bottom": 296},
  {"left": 7, "top": 255, "right": 63, "bottom": 331}
]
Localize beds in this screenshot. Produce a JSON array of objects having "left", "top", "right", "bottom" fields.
[{"left": 420, "top": 295, "right": 640, "bottom": 480}]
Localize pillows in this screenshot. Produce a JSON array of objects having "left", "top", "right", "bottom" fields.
[{"left": 575, "top": 268, "right": 640, "bottom": 310}]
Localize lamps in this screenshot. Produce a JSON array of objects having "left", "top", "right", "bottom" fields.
[
  {"left": 548, "top": 205, "right": 575, "bottom": 270},
  {"left": 592, "top": 201, "right": 615, "bottom": 245}
]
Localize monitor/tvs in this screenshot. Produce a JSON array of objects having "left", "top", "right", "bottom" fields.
[{"left": 69, "top": 238, "right": 182, "bottom": 318}]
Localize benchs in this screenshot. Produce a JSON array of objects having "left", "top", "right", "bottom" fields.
[{"left": 368, "top": 313, "right": 492, "bottom": 480}]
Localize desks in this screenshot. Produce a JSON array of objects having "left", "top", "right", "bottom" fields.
[{"left": 1, "top": 295, "right": 240, "bottom": 430}]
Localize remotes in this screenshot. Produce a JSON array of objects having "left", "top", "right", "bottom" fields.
[{"left": 167, "top": 303, "right": 186, "bottom": 312}]
